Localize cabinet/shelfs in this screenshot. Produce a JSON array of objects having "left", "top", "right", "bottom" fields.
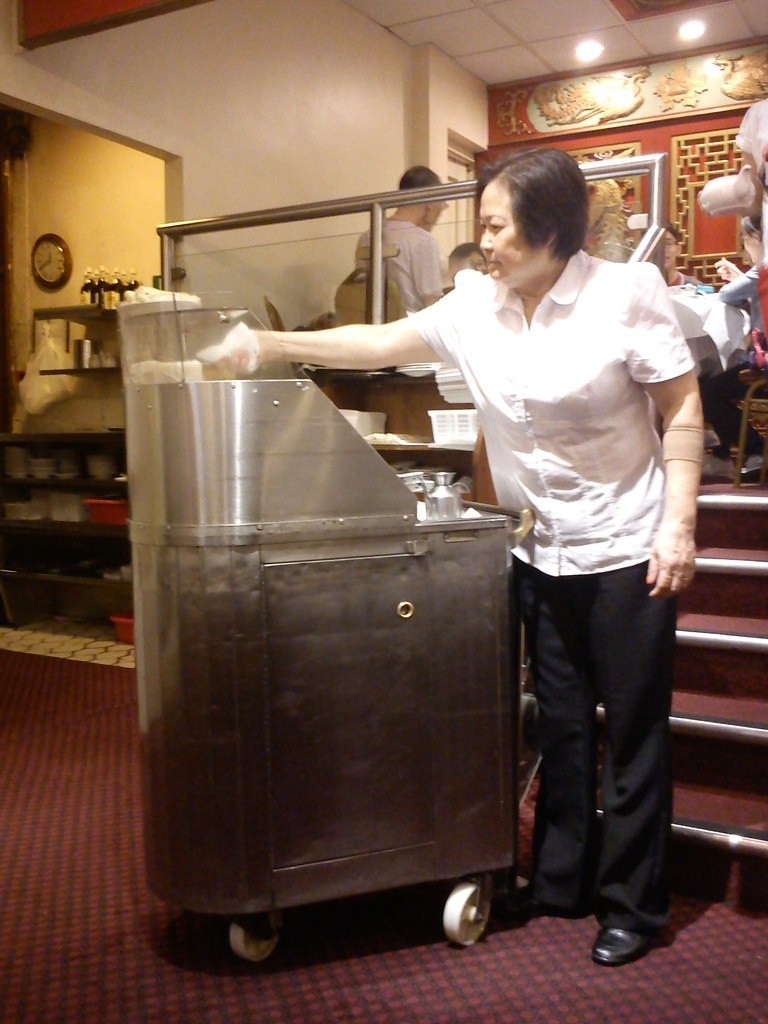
[{"left": 1, "top": 431, "right": 128, "bottom": 616}]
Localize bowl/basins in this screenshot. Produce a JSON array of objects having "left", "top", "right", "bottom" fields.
[
  {"left": 388, "top": 459, "right": 452, "bottom": 493},
  {"left": 107, "top": 608, "right": 135, "bottom": 645},
  {"left": 5, "top": 443, "right": 122, "bottom": 481},
  {"left": 81, "top": 494, "right": 129, "bottom": 525}
]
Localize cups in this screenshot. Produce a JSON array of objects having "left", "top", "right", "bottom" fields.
[
  {"left": 72, "top": 338, "right": 91, "bottom": 370},
  {"left": 450, "top": 476, "right": 474, "bottom": 495}
]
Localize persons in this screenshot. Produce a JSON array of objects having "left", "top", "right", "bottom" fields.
[
  {"left": 640, "top": 224, "right": 705, "bottom": 286},
  {"left": 355, "top": 166, "right": 449, "bottom": 316},
  {"left": 442, "top": 242, "right": 487, "bottom": 295},
  {"left": 197, "top": 147, "right": 705, "bottom": 966},
  {"left": 704, "top": 98, "right": 768, "bottom": 481}
]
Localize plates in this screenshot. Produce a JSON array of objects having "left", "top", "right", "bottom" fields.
[
  {"left": 394, "top": 361, "right": 442, "bottom": 378},
  {"left": 2, "top": 489, "right": 82, "bottom": 522}
]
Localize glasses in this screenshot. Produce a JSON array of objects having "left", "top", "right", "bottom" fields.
[{"left": 434, "top": 200, "right": 449, "bottom": 211}]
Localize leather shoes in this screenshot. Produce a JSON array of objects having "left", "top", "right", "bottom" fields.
[
  {"left": 592, "top": 926, "right": 651, "bottom": 965},
  {"left": 490, "top": 882, "right": 588, "bottom": 922}
]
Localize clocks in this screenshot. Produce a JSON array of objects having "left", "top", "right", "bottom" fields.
[{"left": 30, "top": 232, "right": 74, "bottom": 291}]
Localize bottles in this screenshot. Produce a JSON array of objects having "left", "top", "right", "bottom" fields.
[{"left": 80, "top": 266, "right": 141, "bottom": 311}]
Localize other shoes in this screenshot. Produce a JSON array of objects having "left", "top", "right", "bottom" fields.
[
  {"left": 702, "top": 453, "right": 735, "bottom": 478},
  {"left": 741, "top": 455, "right": 762, "bottom": 473}
]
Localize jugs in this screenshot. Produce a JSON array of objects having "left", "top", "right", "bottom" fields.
[{"left": 412, "top": 470, "right": 472, "bottom": 522}]
